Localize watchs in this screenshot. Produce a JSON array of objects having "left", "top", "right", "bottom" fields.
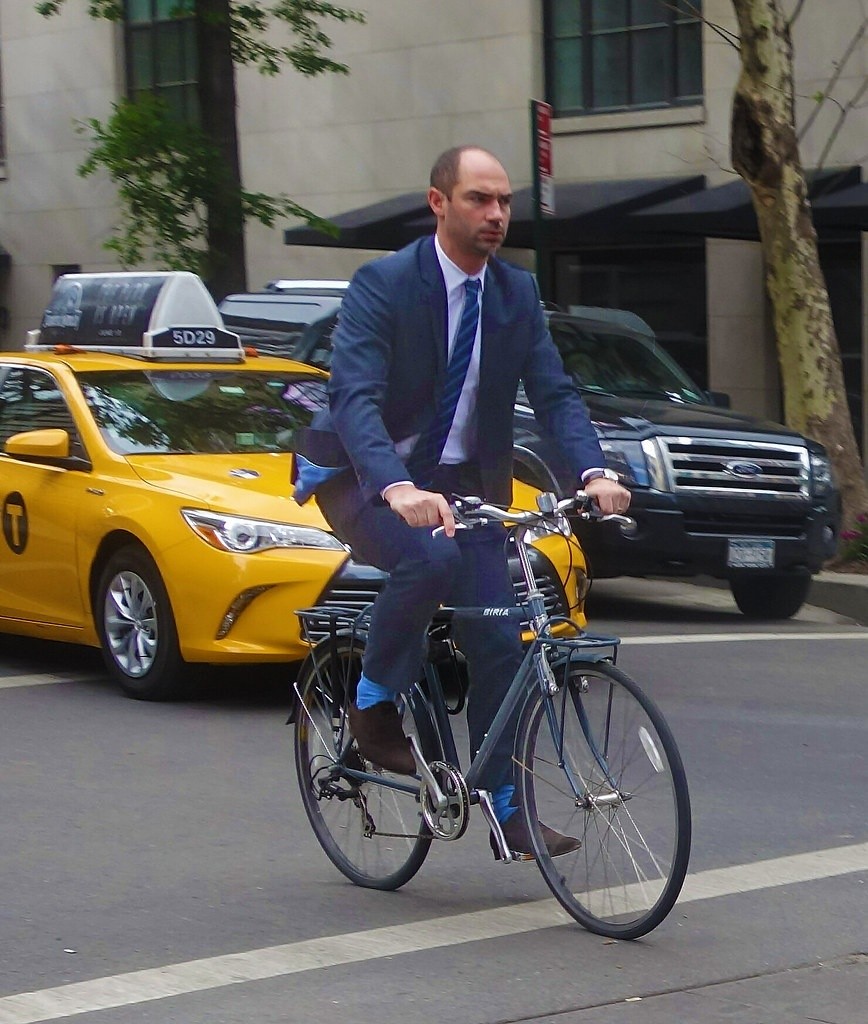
[{"left": 582, "top": 469, "right": 619, "bottom": 486}]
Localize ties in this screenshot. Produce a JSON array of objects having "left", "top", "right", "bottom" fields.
[{"left": 403, "top": 279, "right": 480, "bottom": 489}]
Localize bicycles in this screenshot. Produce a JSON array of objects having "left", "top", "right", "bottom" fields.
[{"left": 286, "top": 492, "right": 692, "bottom": 940}]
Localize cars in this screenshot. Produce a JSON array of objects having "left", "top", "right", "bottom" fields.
[{"left": 0, "top": 271, "right": 592, "bottom": 703}]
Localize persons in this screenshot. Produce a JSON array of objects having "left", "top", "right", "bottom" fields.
[{"left": 292, "top": 145, "right": 631, "bottom": 862}]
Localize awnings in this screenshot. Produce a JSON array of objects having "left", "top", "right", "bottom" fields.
[{"left": 284, "top": 165, "right": 868, "bottom": 253}]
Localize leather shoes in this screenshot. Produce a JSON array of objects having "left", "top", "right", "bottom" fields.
[
  {"left": 489, "top": 809, "right": 583, "bottom": 862},
  {"left": 347, "top": 699, "right": 417, "bottom": 775}
]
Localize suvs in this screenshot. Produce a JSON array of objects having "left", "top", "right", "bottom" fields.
[{"left": 218, "top": 277, "right": 845, "bottom": 620}]
